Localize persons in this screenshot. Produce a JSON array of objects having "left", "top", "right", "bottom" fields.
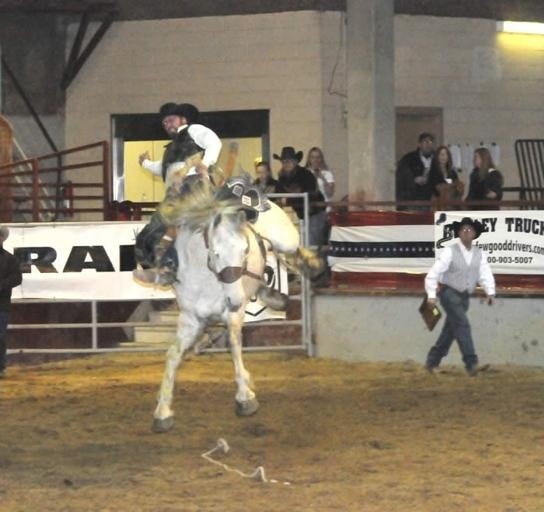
[
  {"left": 130, "top": 105, "right": 334, "bottom": 283},
  {"left": 432, "top": 146, "right": 465, "bottom": 209},
  {"left": 423, "top": 217, "right": 496, "bottom": 376},
  {"left": 273, "top": 147, "right": 331, "bottom": 287},
  {"left": 252, "top": 162, "right": 278, "bottom": 201},
  {"left": 395, "top": 132, "right": 437, "bottom": 211},
  {"left": 468, "top": 148, "right": 503, "bottom": 209},
  {"left": 305, "top": 147, "right": 334, "bottom": 245},
  {"left": 1, "top": 223, "right": 22, "bottom": 371}
]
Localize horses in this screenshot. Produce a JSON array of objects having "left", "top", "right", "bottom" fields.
[{"left": 131, "top": 158, "right": 321, "bottom": 433}]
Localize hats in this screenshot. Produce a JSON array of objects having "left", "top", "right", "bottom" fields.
[
  {"left": 453, "top": 217, "right": 482, "bottom": 238},
  {"left": 273, "top": 146, "right": 304, "bottom": 162},
  {"left": 152, "top": 103, "right": 198, "bottom": 137}
]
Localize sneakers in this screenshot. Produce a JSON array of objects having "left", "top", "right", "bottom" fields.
[
  {"left": 466, "top": 361, "right": 491, "bottom": 378},
  {"left": 133, "top": 269, "right": 176, "bottom": 286},
  {"left": 425, "top": 364, "right": 446, "bottom": 375}
]
